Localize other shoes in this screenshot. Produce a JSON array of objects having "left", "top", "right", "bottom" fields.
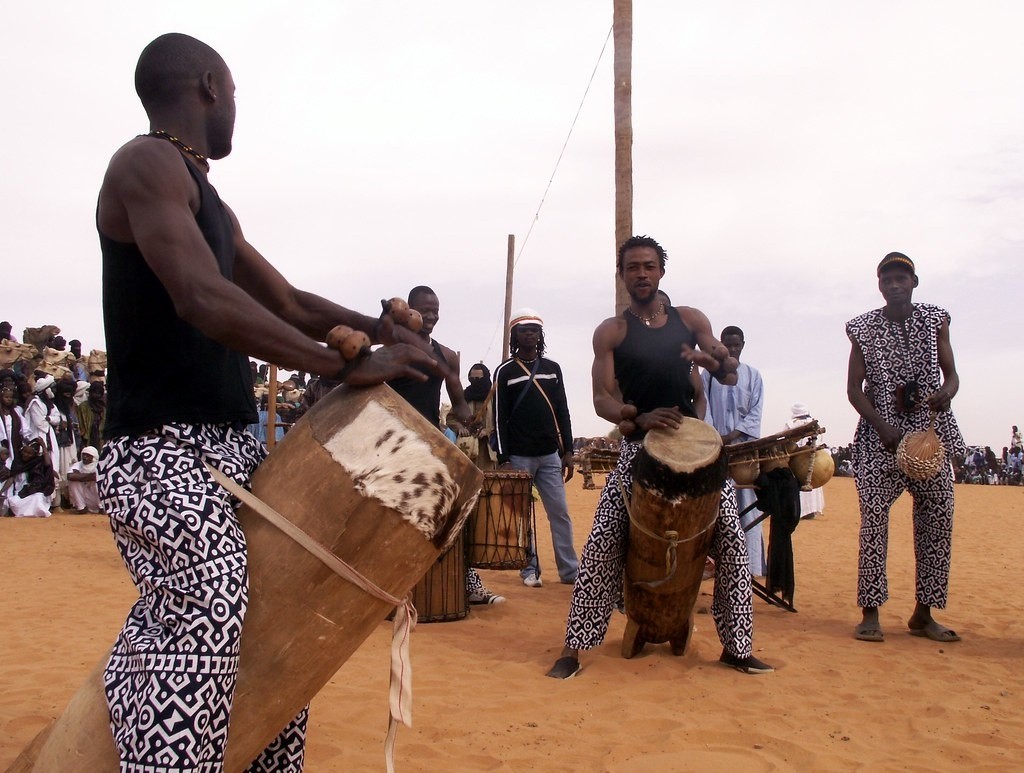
[
  {"left": 99, "top": 508, "right": 104, "bottom": 514},
  {"left": 718, "top": 647, "right": 774, "bottom": 673},
  {"left": 546, "top": 655, "right": 583, "bottom": 679},
  {"left": 55, "top": 506, "right": 64, "bottom": 513},
  {"left": 80, "top": 507, "right": 88, "bottom": 514}
]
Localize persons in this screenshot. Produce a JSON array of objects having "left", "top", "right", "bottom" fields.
[
  {"left": 700, "top": 326, "right": 767, "bottom": 577},
  {"left": 0, "top": 321, "right": 104, "bottom": 517},
  {"left": 573, "top": 437, "right": 620, "bottom": 456},
  {"left": 92, "top": 33, "right": 438, "bottom": 773},
  {"left": 246, "top": 361, "right": 319, "bottom": 448},
  {"left": 546, "top": 237, "right": 775, "bottom": 679},
  {"left": 385, "top": 286, "right": 484, "bottom": 439},
  {"left": 493, "top": 310, "right": 579, "bottom": 587},
  {"left": 845, "top": 252, "right": 966, "bottom": 642},
  {"left": 954, "top": 425, "right": 1024, "bottom": 486},
  {"left": 831, "top": 443, "right": 852, "bottom": 477},
  {"left": 446, "top": 364, "right": 498, "bottom": 471},
  {"left": 784, "top": 403, "right": 825, "bottom": 518}
]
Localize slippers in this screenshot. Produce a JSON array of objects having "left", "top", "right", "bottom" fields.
[
  {"left": 909, "top": 620, "right": 961, "bottom": 641},
  {"left": 855, "top": 624, "right": 884, "bottom": 641}
]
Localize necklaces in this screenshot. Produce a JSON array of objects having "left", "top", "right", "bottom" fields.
[
  {"left": 628, "top": 302, "right": 663, "bottom": 326},
  {"left": 516, "top": 355, "right": 538, "bottom": 363},
  {"left": 150, "top": 131, "right": 210, "bottom": 173}
]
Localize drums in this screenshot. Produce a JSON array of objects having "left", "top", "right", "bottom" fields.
[
  {"left": 385, "top": 528, "right": 474, "bottom": 621},
  {"left": 471, "top": 471, "right": 534, "bottom": 571},
  {"left": 21, "top": 378, "right": 486, "bottom": 773},
  {"left": 623, "top": 415, "right": 728, "bottom": 659}
]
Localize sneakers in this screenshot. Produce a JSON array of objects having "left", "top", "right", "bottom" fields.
[
  {"left": 467, "top": 586, "right": 506, "bottom": 607},
  {"left": 524, "top": 573, "right": 542, "bottom": 587},
  {"left": 562, "top": 579, "right": 576, "bottom": 584}
]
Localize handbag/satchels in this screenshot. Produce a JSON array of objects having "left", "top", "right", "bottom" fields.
[
  {"left": 455, "top": 435, "right": 479, "bottom": 458},
  {"left": 489, "top": 431, "right": 498, "bottom": 451}
]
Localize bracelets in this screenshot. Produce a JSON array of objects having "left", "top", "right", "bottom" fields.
[
  {"left": 373, "top": 319, "right": 386, "bottom": 342},
  {"left": 321, "top": 347, "right": 370, "bottom": 389}
]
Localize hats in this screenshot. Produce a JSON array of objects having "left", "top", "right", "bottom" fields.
[
  {"left": 877, "top": 252, "right": 915, "bottom": 278},
  {"left": 792, "top": 403, "right": 810, "bottom": 417},
  {"left": 35, "top": 375, "right": 54, "bottom": 392},
  {"left": 509, "top": 307, "right": 543, "bottom": 330}
]
